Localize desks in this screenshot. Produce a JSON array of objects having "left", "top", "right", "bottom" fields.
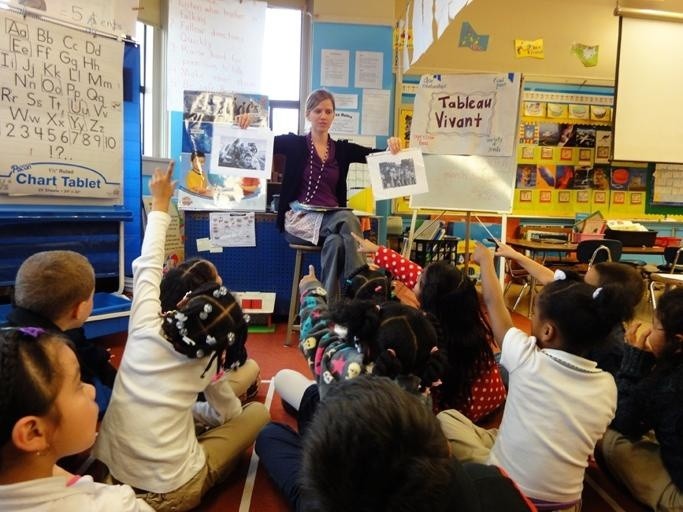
[{"left": 488, "top": 238, "right": 683, "bottom": 319}]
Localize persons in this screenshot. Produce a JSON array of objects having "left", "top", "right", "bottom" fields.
[
  {"left": 236, "top": 90, "right": 402, "bottom": 305},
  {"left": 186, "top": 151, "right": 216, "bottom": 198},
  {"left": 1, "top": 160, "right": 683, "bottom": 511}
]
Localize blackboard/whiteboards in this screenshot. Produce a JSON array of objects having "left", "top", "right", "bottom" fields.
[{"left": 409, "top": 72, "right": 522, "bottom": 214}]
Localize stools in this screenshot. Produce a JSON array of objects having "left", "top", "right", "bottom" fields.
[{"left": 287, "top": 243, "right": 322, "bottom": 348}]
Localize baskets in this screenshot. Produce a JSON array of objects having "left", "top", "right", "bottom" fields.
[{"left": 410, "top": 236, "right": 459, "bottom": 270}]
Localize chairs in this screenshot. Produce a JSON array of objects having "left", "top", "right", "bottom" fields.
[
  {"left": 489, "top": 224, "right": 564, "bottom": 311},
  {"left": 648, "top": 247, "right": 683, "bottom": 309}
]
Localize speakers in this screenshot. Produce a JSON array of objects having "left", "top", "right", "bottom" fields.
[{"left": 346, "top": 272, "right": 389, "bottom": 304}]
